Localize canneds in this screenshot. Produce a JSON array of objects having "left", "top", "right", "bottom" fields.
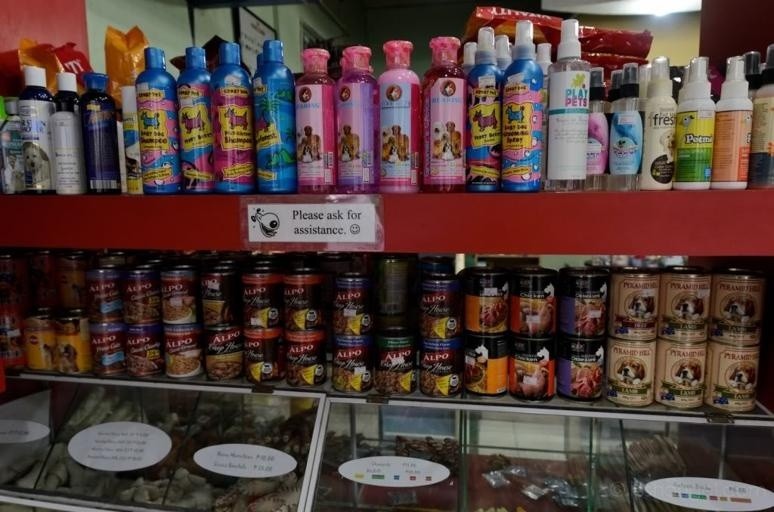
[
  {"left": 243, "top": 324, "right": 286, "bottom": 384},
  {"left": 91, "top": 322, "right": 126, "bottom": 377},
  {"left": 200, "top": 266, "right": 239, "bottom": 327},
  {"left": 19, "top": 305, "right": 57, "bottom": 371},
  {"left": 0, "top": 255, "right": 29, "bottom": 315},
  {"left": 334, "top": 272, "right": 373, "bottom": 337},
  {"left": 86, "top": 255, "right": 121, "bottom": 318},
  {"left": 158, "top": 264, "right": 197, "bottom": 326},
  {"left": 126, "top": 263, "right": 161, "bottom": 327},
  {"left": 52, "top": 307, "right": 90, "bottom": 372},
  {"left": 243, "top": 271, "right": 282, "bottom": 325},
  {"left": 203, "top": 321, "right": 245, "bottom": 380},
  {"left": 375, "top": 258, "right": 760, "bottom": 414},
  {"left": 29, "top": 252, "right": 59, "bottom": 301},
  {"left": 1, "top": 305, "right": 26, "bottom": 369},
  {"left": 123, "top": 324, "right": 166, "bottom": 380},
  {"left": 167, "top": 324, "right": 202, "bottom": 379},
  {"left": 282, "top": 273, "right": 323, "bottom": 332},
  {"left": 284, "top": 331, "right": 327, "bottom": 387},
  {"left": 60, "top": 255, "right": 93, "bottom": 311},
  {"left": 331, "top": 335, "right": 373, "bottom": 393}
]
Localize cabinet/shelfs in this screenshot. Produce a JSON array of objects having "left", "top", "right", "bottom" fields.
[{"left": 0, "top": 185, "right": 774, "bottom": 510}]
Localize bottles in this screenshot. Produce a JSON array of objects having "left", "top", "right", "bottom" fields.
[{"left": 0, "top": 17, "right": 774, "bottom": 200}]
